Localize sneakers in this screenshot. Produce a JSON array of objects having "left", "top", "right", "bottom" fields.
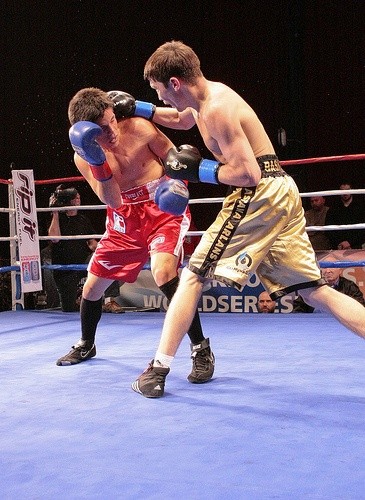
[
  {"left": 56, "top": 339, "right": 96, "bottom": 366},
  {"left": 131, "top": 359, "right": 170, "bottom": 397},
  {"left": 102, "top": 300, "right": 123, "bottom": 313},
  {"left": 187, "top": 337, "right": 215, "bottom": 383}
]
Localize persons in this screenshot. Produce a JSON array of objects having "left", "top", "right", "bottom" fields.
[
  {"left": 55, "top": 88, "right": 216, "bottom": 384},
  {"left": 0, "top": 184, "right": 365, "bottom": 315},
  {"left": 105, "top": 40, "right": 365, "bottom": 398}
]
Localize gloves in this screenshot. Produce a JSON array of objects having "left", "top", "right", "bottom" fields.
[
  {"left": 69, "top": 121, "right": 113, "bottom": 182},
  {"left": 165, "top": 145, "right": 222, "bottom": 185},
  {"left": 155, "top": 179, "right": 190, "bottom": 215},
  {"left": 106, "top": 90, "right": 156, "bottom": 122}
]
